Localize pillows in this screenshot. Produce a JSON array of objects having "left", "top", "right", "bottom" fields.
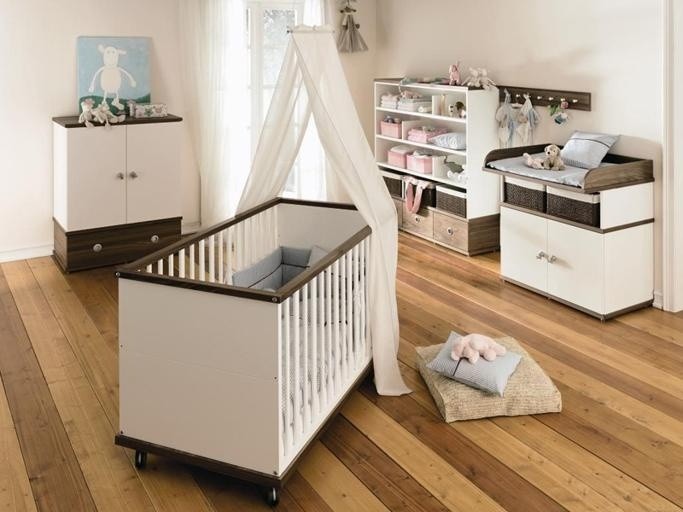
[
  {"left": 307, "top": 246, "right": 365, "bottom": 300},
  {"left": 415, "top": 329, "right": 561, "bottom": 422},
  {"left": 557, "top": 131, "right": 620, "bottom": 169}
]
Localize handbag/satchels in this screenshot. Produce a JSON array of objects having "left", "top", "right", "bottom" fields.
[{"left": 404, "top": 177, "right": 432, "bottom": 214}]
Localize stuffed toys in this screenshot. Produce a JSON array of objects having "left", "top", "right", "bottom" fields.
[
  {"left": 523, "top": 152, "right": 545, "bottom": 169},
  {"left": 448, "top": 64, "right": 496, "bottom": 92},
  {"left": 78, "top": 97, "right": 144, "bottom": 131},
  {"left": 448, "top": 101, "right": 466, "bottom": 119},
  {"left": 543, "top": 144, "right": 565, "bottom": 170},
  {"left": 451, "top": 332, "right": 506, "bottom": 363}
]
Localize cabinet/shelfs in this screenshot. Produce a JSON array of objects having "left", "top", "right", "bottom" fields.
[
  {"left": 500, "top": 174, "right": 656, "bottom": 320},
  {"left": 374, "top": 79, "right": 500, "bottom": 256},
  {"left": 52, "top": 113, "right": 191, "bottom": 274}
]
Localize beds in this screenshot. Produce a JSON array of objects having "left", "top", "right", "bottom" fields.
[{"left": 114, "top": 197, "right": 399, "bottom": 507}]
